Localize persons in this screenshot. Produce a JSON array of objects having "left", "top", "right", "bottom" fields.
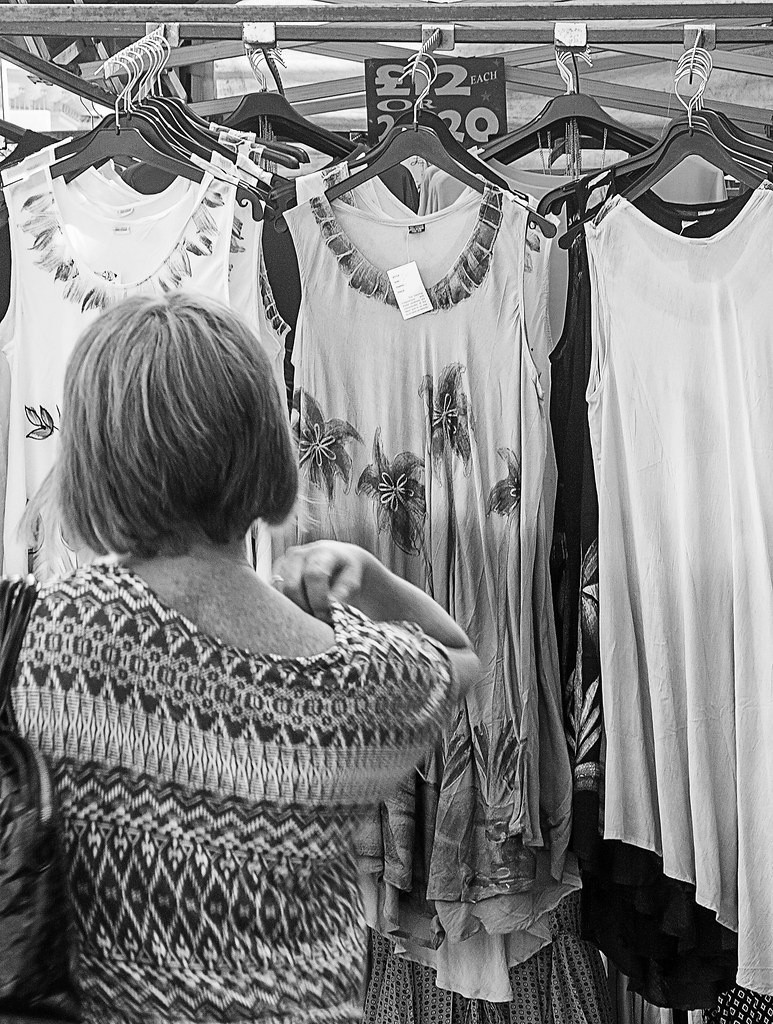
[{"left": 4, "top": 293, "right": 476, "bottom": 1023}]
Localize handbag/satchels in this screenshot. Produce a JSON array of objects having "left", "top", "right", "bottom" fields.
[{"left": 0, "top": 581, "right": 78, "bottom": 1024}]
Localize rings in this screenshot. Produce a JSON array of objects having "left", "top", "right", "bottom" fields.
[{"left": 269, "top": 573, "right": 285, "bottom": 586}]
